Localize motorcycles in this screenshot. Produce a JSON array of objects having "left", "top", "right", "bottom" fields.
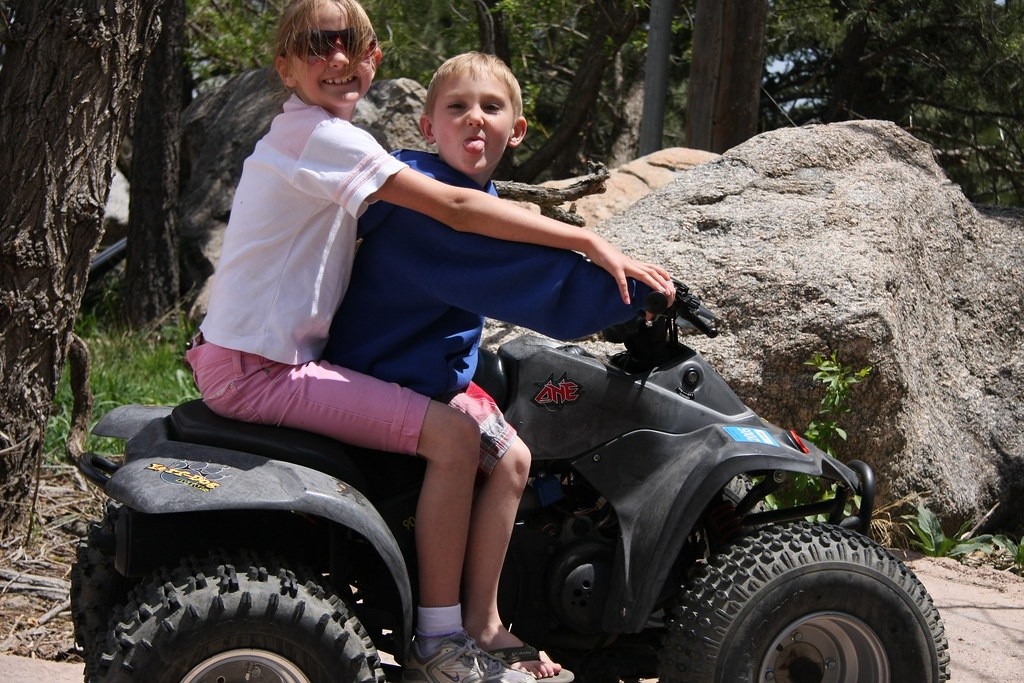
[{"left": 69, "top": 278, "right": 954, "bottom": 682}]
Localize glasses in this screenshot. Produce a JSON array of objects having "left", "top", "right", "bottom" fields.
[{"left": 280, "top": 26, "right": 377, "bottom": 56}]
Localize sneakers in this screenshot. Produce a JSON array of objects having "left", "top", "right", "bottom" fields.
[{"left": 403, "top": 630, "right": 536, "bottom": 683}]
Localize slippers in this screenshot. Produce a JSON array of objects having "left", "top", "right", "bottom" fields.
[{"left": 485, "top": 639, "right": 575, "bottom": 683}]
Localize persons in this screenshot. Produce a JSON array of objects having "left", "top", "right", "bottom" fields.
[
  {"left": 321, "top": 51, "right": 675, "bottom": 683},
  {"left": 185, "top": 1, "right": 673, "bottom": 682}
]
